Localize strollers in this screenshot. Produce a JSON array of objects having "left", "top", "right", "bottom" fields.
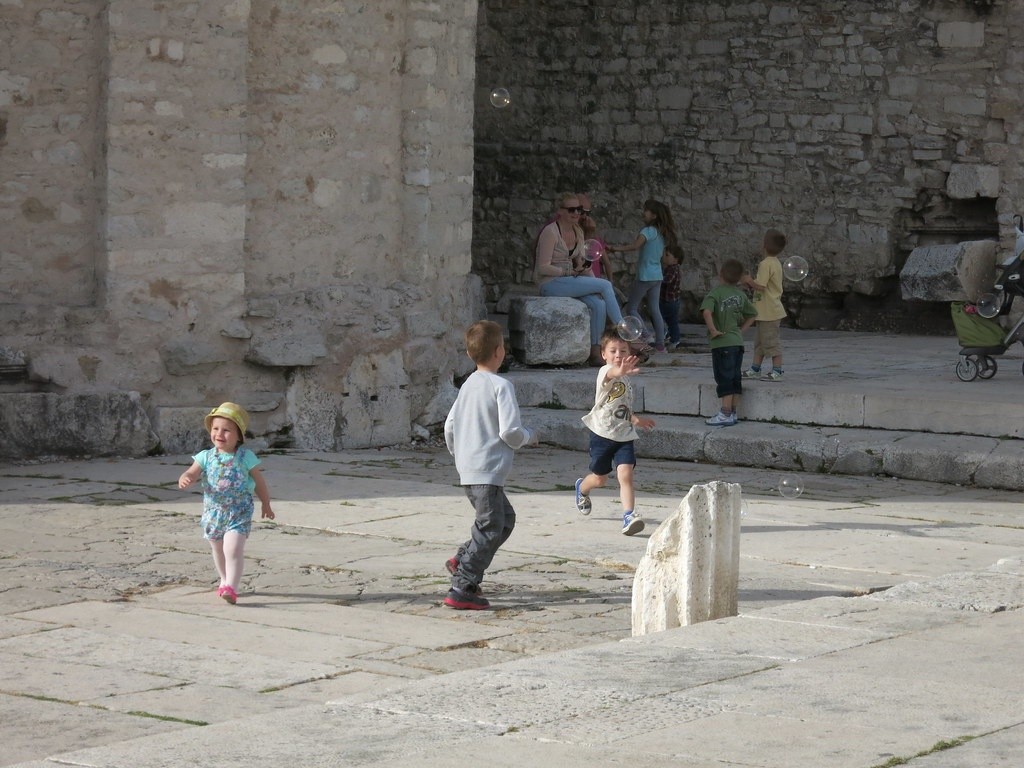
[{"left": 950, "top": 212, "right": 1024, "bottom": 382}]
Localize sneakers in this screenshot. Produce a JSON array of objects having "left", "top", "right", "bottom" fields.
[
  {"left": 760, "top": 369, "right": 783, "bottom": 382},
  {"left": 446, "top": 555, "right": 483, "bottom": 596},
  {"left": 666, "top": 342, "right": 681, "bottom": 350},
  {"left": 646, "top": 348, "right": 668, "bottom": 355},
  {"left": 444, "top": 588, "right": 489, "bottom": 610},
  {"left": 575, "top": 477, "right": 592, "bottom": 516},
  {"left": 622, "top": 511, "right": 645, "bottom": 536},
  {"left": 704, "top": 411, "right": 733, "bottom": 426},
  {"left": 741, "top": 368, "right": 761, "bottom": 379},
  {"left": 733, "top": 413, "right": 738, "bottom": 422}
]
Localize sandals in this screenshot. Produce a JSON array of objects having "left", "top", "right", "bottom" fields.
[{"left": 631, "top": 341, "right": 653, "bottom": 351}]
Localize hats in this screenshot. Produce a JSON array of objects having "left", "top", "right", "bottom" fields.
[{"left": 203, "top": 402, "right": 249, "bottom": 444}]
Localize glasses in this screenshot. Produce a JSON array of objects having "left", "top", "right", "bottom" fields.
[
  {"left": 561, "top": 205, "right": 584, "bottom": 213},
  {"left": 581, "top": 209, "right": 592, "bottom": 216}
]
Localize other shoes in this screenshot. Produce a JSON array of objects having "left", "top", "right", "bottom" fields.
[{"left": 218, "top": 585, "right": 237, "bottom": 605}]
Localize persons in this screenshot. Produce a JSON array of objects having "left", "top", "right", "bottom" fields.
[
  {"left": 533, "top": 192, "right": 653, "bottom": 367},
  {"left": 179, "top": 402, "right": 275, "bottom": 604},
  {"left": 606, "top": 200, "right": 678, "bottom": 353},
  {"left": 539, "top": 193, "right": 596, "bottom": 241},
  {"left": 698, "top": 259, "right": 758, "bottom": 426},
  {"left": 586, "top": 233, "right": 613, "bottom": 281},
  {"left": 575, "top": 324, "right": 656, "bottom": 536},
  {"left": 444, "top": 320, "right": 538, "bottom": 609},
  {"left": 741, "top": 229, "right": 788, "bottom": 380},
  {"left": 660, "top": 245, "right": 685, "bottom": 348}
]
